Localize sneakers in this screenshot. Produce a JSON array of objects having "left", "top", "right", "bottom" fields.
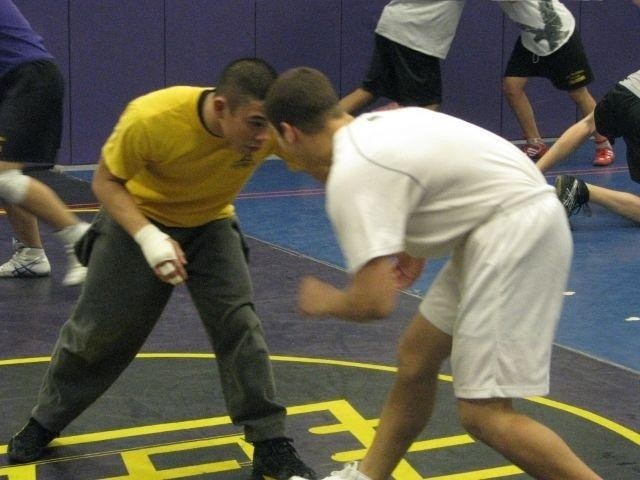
[
  {"left": 253, "top": 437, "right": 316, "bottom": 480},
  {"left": 0, "top": 243, "right": 51, "bottom": 277},
  {"left": 520, "top": 143, "right": 551, "bottom": 159},
  {"left": 51, "top": 224, "right": 91, "bottom": 286},
  {"left": 7, "top": 415, "right": 59, "bottom": 463},
  {"left": 554, "top": 175, "right": 589, "bottom": 217},
  {"left": 591, "top": 135, "right": 616, "bottom": 166},
  {"left": 321, "top": 460, "right": 372, "bottom": 480}
]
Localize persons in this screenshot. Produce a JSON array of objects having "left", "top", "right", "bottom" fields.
[
  {"left": 6, "top": 58, "right": 316, "bottom": 479},
  {"left": 263, "top": 66, "right": 601, "bottom": 480},
  {"left": 496, "top": 1, "right": 616, "bottom": 167},
  {"left": 535, "top": 69, "right": 640, "bottom": 231},
  {"left": 338, "top": 1, "right": 465, "bottom": 115},
  {"left": 0, "top": 1, "right": 93, "bottom": 286}
]
